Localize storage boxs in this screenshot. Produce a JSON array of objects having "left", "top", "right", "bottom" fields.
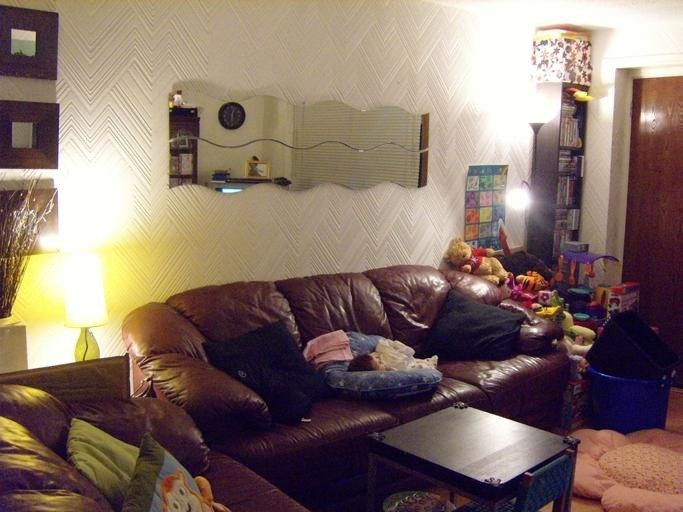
[{"left": 533, "top": 29, "right": 593, "bottom": 86}]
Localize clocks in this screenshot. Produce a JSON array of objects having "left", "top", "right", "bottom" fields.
[{"left": 216, "top": 101, "right": 246, "bottom": 130}]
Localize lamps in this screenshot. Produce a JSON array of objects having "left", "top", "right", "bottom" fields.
[
  {"left": 520, "top": 91, "right": 560, "bottom": 260},
  {"left": 506, "top": 95, "right": 560, "bottom": 232},
  {"left": 60, "top": 249, "right": 108, "bottom": 362}
]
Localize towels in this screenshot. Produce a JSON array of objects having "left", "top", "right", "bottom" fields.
[{"left": 302, "top": 328, "right": 354, "bottom": 365}]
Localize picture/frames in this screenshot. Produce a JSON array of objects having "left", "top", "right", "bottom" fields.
[{"left": 245, "top": 160, "right": 270, "bottom": 180}]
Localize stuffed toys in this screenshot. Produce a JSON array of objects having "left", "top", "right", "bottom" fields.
[{"left": 441, "top": 236, "right": 513, "bottom": 286}]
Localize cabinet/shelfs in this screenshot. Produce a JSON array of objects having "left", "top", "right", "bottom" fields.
[{"left": 527, "top": 79, "right": 589, "bottom": 273}]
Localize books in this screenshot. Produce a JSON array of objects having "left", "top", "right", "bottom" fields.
[
  {"left": 552, "top": 209, "right": 579, "bottom": 259},
  {"left": 560, "top": 91, "right": 582, "bottom": 147},
  {"left": 557, "top": 176, "right": 574, "bottom": 206},
  {"left": 558, "top": 150, "right": 584, "bottom": 178}
]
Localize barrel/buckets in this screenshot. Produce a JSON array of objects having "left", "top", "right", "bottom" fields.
[{"left": 583, "top": 369, "right": 675, "bottom": 433}]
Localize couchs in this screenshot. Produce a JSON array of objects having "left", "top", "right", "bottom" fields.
[
  {"left": 1, "top": 352, "right": 304, "bottom": 510},
  {"left": 120, "top": 264, "right": 570, "bottom": 500}
]
